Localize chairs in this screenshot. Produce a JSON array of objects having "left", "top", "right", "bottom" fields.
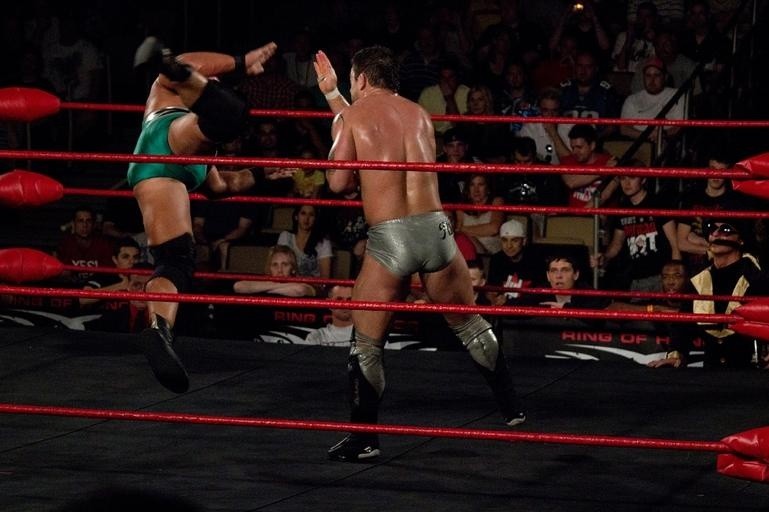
[
  {"left": 506, "top": 216, "right": 527, "bottom": 238},
  {"left": 228, "top": 246, "right": 267, "bottom": 274},
  {"left": 533, "top": 217, "right": 594, "bottom": 254},
  {"left": 603, "top": 141, "right": 652, "bottom": 168},
  {"left": 331, "top": 251, "right": 351, "bottom": 280},
  {"left": 261, "top": 207, "right": 295, "bottom": 233}
]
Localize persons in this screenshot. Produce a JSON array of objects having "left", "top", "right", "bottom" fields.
[
  {"left": 49, "top": 2, "right": 769, "bottom": 368},
  {"left": 314, "top": 45, "right": 527, "bottom": 465},
  {"left": 127, "top": 36, "right": 278, "bottom": 394}
]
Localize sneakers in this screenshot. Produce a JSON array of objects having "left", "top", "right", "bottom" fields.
[
  {"left": 136, "top": 314, "right": 193, "bottom": 395},
  {"left": 326, "top": 435, "right": 382, "bottom": 463},
  {"left": 132, "top": 35, "right": 177, "bottom": 77},
  {"left": 502, "top": 401, "right": 526, "bottom": 427}
]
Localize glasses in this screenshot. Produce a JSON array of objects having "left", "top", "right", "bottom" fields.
[{"left": 705, "top": 222, "right": 738, "bottom": 239}]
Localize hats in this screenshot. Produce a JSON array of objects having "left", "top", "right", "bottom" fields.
[
  {"left": 497, "top": 217, "right": 527, "bottom": 239},
  {"left": 443, "top": 126, "right": 468, "bottom": 145},
  {"left": 642, "top": 56, "right": 665, "bottom": 70}
]
